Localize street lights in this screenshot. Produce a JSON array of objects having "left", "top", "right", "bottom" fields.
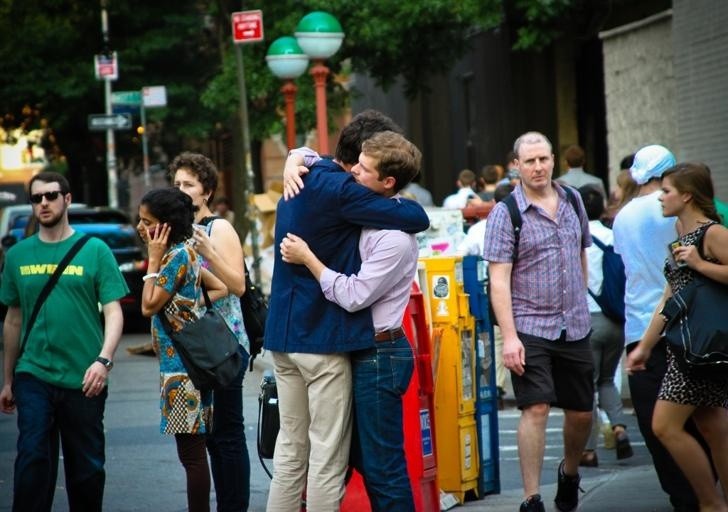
[
  {"left": 293, "top": 11, "right": 346, "bottom": 157},
  {"left": 266, "top": 36, "right": 313, "bottom": 152}
]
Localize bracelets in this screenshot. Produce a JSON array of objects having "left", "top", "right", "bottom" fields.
[{"left": 142, "top": 272, "right": 159, "bottom": 281}]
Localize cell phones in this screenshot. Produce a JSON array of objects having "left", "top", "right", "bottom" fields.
[
  {"left": 149, "top": 223, "right": 162, "bottom": 238},
  {"left": 668, "top": 239, "right": 687, "bottom": 268}
]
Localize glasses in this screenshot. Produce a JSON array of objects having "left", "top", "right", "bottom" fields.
[{"left": 29, "top": 188, "right": 62, "bottom": 203}]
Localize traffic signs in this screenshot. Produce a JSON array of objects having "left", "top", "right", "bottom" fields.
[
  {"left": 110, "top": 91, "right": 142, "bottom": 104},
  {"left": 89, "top": 113, "right": 130, "bottom": 130}
]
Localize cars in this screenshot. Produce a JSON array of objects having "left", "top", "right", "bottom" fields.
[{"left": 0, "top": 201, "right": 149, "bottom": 331}]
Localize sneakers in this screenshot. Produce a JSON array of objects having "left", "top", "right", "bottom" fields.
[
  {"left": 553, "top": 457, "right": 581, "bottom": 512},
  {"left": 518, "top": 494, "right": 546, "bottom": 512}
]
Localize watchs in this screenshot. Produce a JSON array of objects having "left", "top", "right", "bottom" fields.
[{"left": 95, "top": 356, "right": 114, "bottom": 371}]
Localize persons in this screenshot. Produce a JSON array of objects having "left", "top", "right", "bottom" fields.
[
  {"left": 267, "top": 109, "right": 427, "bottom": 511},
  {"left": 280, "top": 131, "right": 418, "bottom": 512},
  {"left": 134, "top": 187, "right": 228, "bottom": 511},
  {"left": 405, "top": 141, "right": 728, "bottom": 469},
  {"left": 486, "top": 130, "right": 595, "bottom": 511},
  {"left": 624, "top": 165, "right": 728, "bottom": 511},
  {"left": 0, "top": 170, "right": 125, "bottom": 511},
  {"left": 614, "top": 145, "right": 719, "bottom": 511},
  {"left": 171, "top": 153, "right": 250, "bottom": 511}
]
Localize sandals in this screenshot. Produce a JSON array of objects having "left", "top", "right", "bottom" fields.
[
  {"left": 613, "top": 430, "right": 633, "bottom": 461},
  {"left": 578, "top": 450, "right": 599, "bottom": 468}
]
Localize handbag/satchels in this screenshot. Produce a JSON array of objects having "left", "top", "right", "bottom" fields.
[
  {"left": 661, "top": 268, "right": 728, "bottom": 371},
  {"left": 259, "top": 372, "right": 357, "bottom": 461},
  {"left": 169, "top": 307, "right": 250, "bottom": 391}
]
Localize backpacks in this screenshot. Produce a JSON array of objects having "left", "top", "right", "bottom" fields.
[
  {"left": 598, "top": 242, "right": 627, "bottom": 322},
  {"left": 238, "top": 258, "right": 269, "bottom": 354}
]
